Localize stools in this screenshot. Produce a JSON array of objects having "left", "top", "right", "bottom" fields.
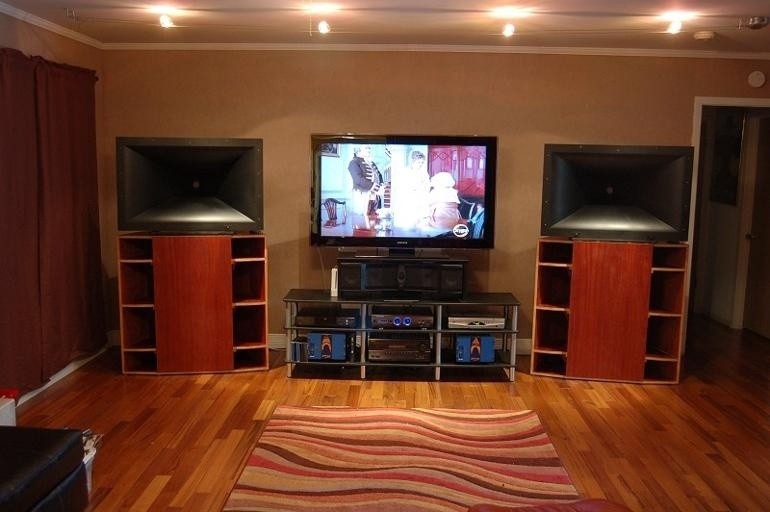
[{"left": 1, "top": 425, "right": 91, "bottom": 512}]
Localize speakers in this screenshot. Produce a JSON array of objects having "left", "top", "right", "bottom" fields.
[
  {"left": 116, "top": 136, "right": 264, "bottom": 232},
  {"left": 540, "top": 142, "right": 694, "bottom": 242}
]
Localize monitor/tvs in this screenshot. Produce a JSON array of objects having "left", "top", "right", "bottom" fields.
[{"left": 308, "top": 133, "right": 498, "bottom": 262}]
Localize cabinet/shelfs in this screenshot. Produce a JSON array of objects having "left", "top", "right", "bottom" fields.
[
  {"left": 531, "top": 235, "right": 690, "bottom": 385},
  {"left": 111, "top": 231, "right": 271, "bottom": 379},
  {"left": 279, "top": 287, "right": 521, "bottom": 387}
]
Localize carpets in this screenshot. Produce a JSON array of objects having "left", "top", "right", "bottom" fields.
[{"left": 222, "top": 403, "right": 582, "bottom": 511}]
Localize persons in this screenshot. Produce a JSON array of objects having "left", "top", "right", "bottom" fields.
[
  {"left": 412, "top": 151, "right": 461, "bottom": 228},
  {"left": 348, "top": 144, "right": 384, "bottom": 230}
]
